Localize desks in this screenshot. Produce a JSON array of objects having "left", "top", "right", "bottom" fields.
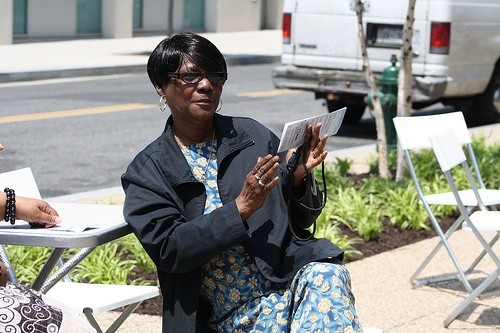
[{"left": 0, "top": 202, "right": 134, "bottom": 297}]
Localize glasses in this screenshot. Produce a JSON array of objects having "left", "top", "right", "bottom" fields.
[{"left": 167, "top": 72, "right": 227, "bottom": 85}]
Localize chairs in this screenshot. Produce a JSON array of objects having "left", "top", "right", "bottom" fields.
[{"left": 392, "top": 111, "right": 500, "bottom": 326}]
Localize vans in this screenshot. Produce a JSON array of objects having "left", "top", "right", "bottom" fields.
[{"left": 270, "top": 1, "right": 500, "bottom": 122}]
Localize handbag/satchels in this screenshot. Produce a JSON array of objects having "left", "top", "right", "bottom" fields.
[{"left": 287, "top": 146, "right": 322, "bottom": 229}]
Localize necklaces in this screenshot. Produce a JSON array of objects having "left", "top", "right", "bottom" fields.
[{"left": 172, "top": 124, "right": 215, "bottom": 183}]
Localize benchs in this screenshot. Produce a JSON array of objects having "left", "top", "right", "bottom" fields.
[{"left": 38, "top": 282, "right": 159, "bottom": 333}]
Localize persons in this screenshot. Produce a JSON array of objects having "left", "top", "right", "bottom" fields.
[
  {"left": 121, "top": 31, "right": 360, "bottom": 333},
  {"left": 0, "top": 143, "right": 61, "bottom": 333}
]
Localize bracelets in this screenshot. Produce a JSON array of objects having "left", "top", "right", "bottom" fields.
[
  {"left": 4, "top": 187, "right": 11, "bottom": 222},
  {"left": 8, "top": 189, "right": 16, "bottom": 224}
]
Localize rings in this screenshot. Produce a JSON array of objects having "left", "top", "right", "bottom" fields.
[
  {"left": 254, "top": 174, "right": 261, "bottom": 180},
  {"left": 258, "top": 180, "right": 265, "bottom": 187}
]
